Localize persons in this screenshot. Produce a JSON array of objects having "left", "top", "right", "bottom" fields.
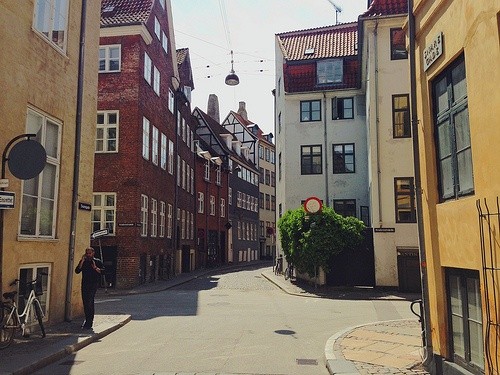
[{"left": 75, "top": 246, "right": 105, "bottom": 328}]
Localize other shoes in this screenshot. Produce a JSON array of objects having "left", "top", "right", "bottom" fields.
[{"left": 83, "top": 322, "right": 93, "bottom": 329}]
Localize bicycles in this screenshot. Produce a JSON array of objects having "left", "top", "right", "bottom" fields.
[
  {"left": 0, "top": 272, "right": 49, "bottom": 351},
  {"left": 274, "top": 260, "right": 280, "bottom": 276},
  {"left": 284, "top": 261, "right": 294, "bottom": 283}
]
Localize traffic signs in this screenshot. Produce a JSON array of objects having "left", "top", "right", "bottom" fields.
[{"left": 91, "top": 228, "right": 109, "bottom": 240}]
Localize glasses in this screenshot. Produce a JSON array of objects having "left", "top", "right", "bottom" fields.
[{"left": 86, "top": 251, "right": 94, "bottom": 254}]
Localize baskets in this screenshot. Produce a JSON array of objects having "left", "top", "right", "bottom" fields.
[{"left": 23, "top": 282, "right": 43, "bottom": 300}]
